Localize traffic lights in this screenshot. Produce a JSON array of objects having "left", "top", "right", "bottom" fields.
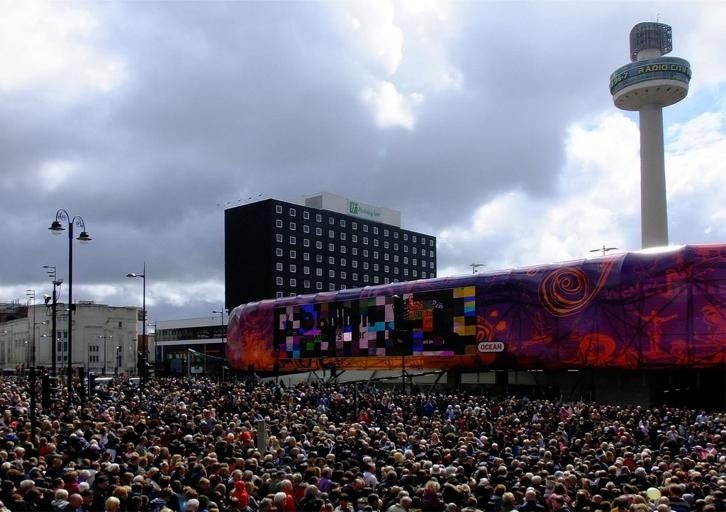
[{"left": 43, "top": 375, "right": 59, "bottom": 409}]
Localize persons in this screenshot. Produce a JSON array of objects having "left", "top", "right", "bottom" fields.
[{"left": 0, "top": 359, "right": 726, "bottom": 512}]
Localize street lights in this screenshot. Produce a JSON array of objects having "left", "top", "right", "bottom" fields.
[
  {"left": 212, "top": 306, "right": 229, "bottom": 344},
  {"left": 3, "top": 270, "right": 69, "bottom": 373},
  {"left": 126, "top": 261, "right": 149, "bottom": 382},
  {"left": 116, "top": 346, "right": 120, "bottom": 368},
  {"left": 48, "top": 209, "right": 92, "bottom": 400},
  {"left": 146, "top": 322, "right": 158, "bottom": 360},
  {"left": 98, "top": 335, "right": 112, "bottom": 374}
]
